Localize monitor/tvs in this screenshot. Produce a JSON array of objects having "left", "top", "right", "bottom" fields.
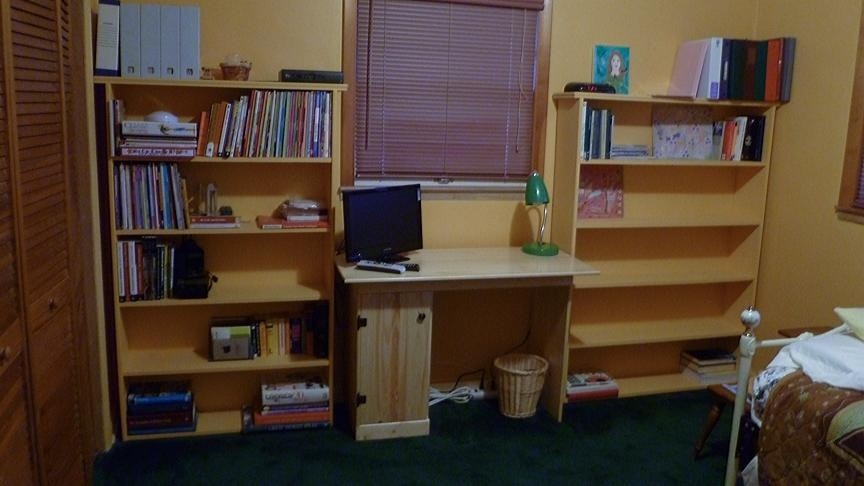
[{"left": 342, "top": 182, "right": 423, "bottom": 262}]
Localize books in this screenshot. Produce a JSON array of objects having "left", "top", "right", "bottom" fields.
[
  {"left": 279, "top": 69, "right": 343, "bottom": 84},
  {"left": 580, "top": 35, "right": 796, "bottom": 163},
  {"left": 565, "top": 372, "right": 618, "bottom": 402},
  {"left": 240, "top": 369, "right": 330, "bottom": 431},
  {"left": 258, "top": 199, "right": 329, "bottom": 228},
  {"left": 116, "top": 239, "right": 174, "bottom": 302},
  {"left": 108, "top": 89, "right": 331, "bottom": 159},
  {"left": 113, "top": 161, "right": 240, "bottom": 229},
  {"left": 211, "top": 314, "right": 307, "bottom": 360},
  {"left": 125, "top": 380, "right": 199, "bottom": 434},
  {"left": 678, "top": 348, "right": 737, "bottom": 386}
]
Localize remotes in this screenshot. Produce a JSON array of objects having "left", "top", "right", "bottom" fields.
[
  {"left": 399, "top": 263, "right": 420, "bottom": 272},
  {"left": 358, "top": 258, "right": 405, "bottom": 275}
]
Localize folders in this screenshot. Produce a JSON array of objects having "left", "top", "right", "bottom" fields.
[
  {"left": 95, "top": 0, "right": 121, "bottom": 76},
  {"left": 697, "top": 37, "right": 798, "bottom": 101},
  {"left": 120, "top": 4, "right": 201, "bottom": 81}
]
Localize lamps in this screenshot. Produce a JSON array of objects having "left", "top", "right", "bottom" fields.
[{"left": 521, "top": 169, "right": 563, "bottom": 257}]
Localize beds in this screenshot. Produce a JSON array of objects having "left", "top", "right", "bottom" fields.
[{"left": 721, "top": 303, "right": 862, "bottom": 486}]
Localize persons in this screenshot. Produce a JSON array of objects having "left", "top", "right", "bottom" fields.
[{"left": 605, "top": 52, "right": 626, "bottom": 92}]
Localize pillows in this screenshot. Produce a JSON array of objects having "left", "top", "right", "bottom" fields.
[
  {"left": 788, "top": 324, "right": 863, "bottom": 394},
  {"left": 834, "top": 306, "right": 863, "bottom": 340}
]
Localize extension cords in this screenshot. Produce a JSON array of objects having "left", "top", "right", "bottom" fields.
[{"left": 469, "top": 387, "right": 495, "bottom": 401}]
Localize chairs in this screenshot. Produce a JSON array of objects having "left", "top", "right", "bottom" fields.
[{"left": 690, "top": 323, "right": 834, "bottom": 463}]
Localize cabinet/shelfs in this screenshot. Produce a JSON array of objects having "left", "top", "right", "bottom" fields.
[
  {"left": 526, "top": 91, "right": 777, "bottom": 422},
  {"left": 0, "top": 0, "right": 103, "bottom": 486},
  {"left": 93, "top": 76, "right": 348, "bottom": 445}
]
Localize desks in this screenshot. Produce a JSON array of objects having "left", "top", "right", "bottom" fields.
[{"left": 332, "top": 243, "right": 600, "bottom": 441}]
